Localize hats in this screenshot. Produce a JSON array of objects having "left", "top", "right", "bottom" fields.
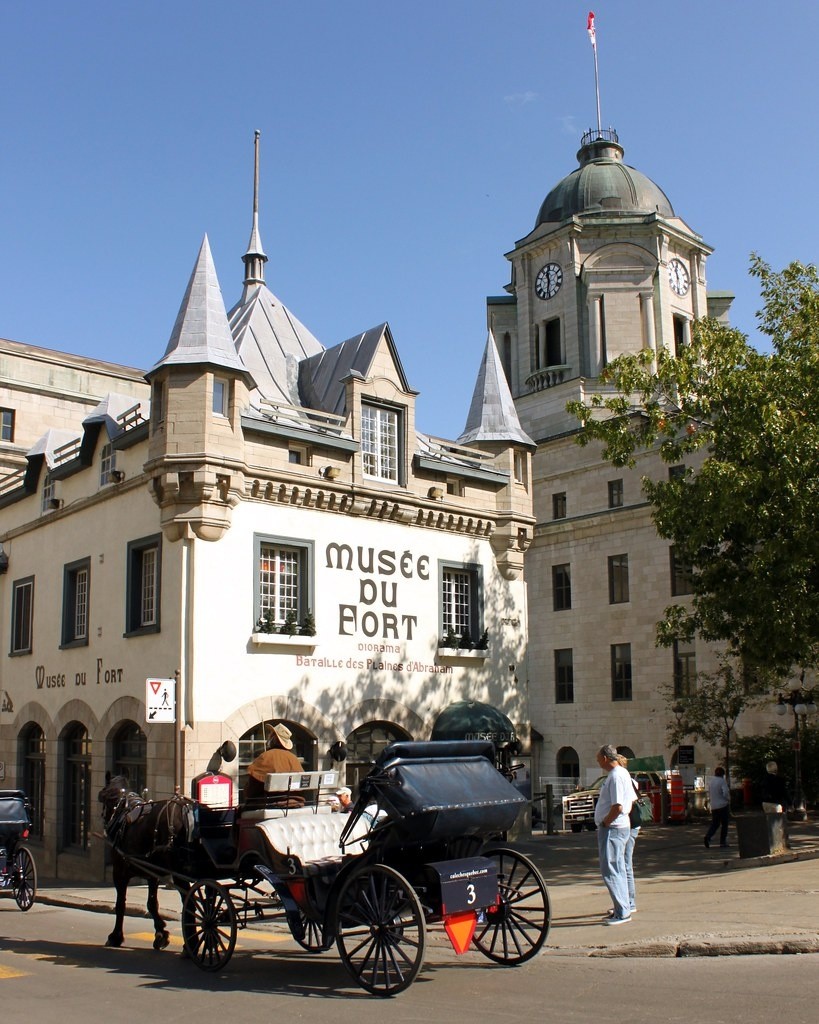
[
  {"left": 335, "top": 786, "right": 352, "bottom": 795},
  {"left": 265, "top": 723, "right": 293, "bottom": 749}
]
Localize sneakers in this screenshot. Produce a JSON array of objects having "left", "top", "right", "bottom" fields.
[
  {"left": 607, "top": 908, "right": 636, "bottom": 914},
  {"left": 602, "top": 915, "right": 631, "bottom": 925}
]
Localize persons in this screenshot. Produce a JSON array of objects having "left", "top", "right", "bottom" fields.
[
  {"left": 595, "top": 744, "right": 643, "bottom": 926},
  {"left": 764, "top": 761, "right": 795, "bottom": 849},
  {"left": 242, "top": 723, "right": 305, "bottom": 809},
  {"left": 327, "top": 787, "right": 354, "bottom": 814},
  {"left": 704, "top": 767, "right": 730, "bottom": 848}
]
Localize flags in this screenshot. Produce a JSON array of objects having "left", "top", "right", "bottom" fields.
[{"left": 587, "top": 11, "right": 595, "bottom": 47}]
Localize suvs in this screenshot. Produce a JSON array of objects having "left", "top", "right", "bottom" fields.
[{"left": 562, "top": 771, "right": 671, "bottom": 832}]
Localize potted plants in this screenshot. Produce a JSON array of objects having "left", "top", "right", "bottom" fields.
[
  {"left": 437, "top": 626, "right": 491, "bottom": 659},
  {"left": 253, "top": 607, "right": 322, "bottom": 646}
]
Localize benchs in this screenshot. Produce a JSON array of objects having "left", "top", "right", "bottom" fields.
[
  {"left": 239, "top": 770, "right": 339, "bottom": 819},
  {"left": 255, "top": 812, "right": 373, "bottom": 879}
]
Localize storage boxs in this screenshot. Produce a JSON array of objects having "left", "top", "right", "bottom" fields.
[{"left": 412, "top": 856, "right": 499, "bottom": 918}]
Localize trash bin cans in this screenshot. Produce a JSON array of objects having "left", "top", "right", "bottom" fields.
[{"left": 506, "top": 771, "right": 532, "bottom": 841}]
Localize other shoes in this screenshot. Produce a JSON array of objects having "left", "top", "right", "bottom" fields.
[
  {"left": 704, "top": 837, "right": 709, "bottom": 848},
  {"left": 720, "top": 843, "right": 729, "bottom": 847}
]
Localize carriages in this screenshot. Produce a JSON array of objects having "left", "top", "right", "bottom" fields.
[{"left": 98, "top": 740, "right": 551, "bottom": 996}]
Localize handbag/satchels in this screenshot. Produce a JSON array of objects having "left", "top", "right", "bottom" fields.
[{"left": 630, "top": 796, "right": 653, "bottom": 829}]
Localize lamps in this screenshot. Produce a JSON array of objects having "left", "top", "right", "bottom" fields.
[
  {"left": 107, "top": 470, "right": 125, "bottom": 483},
  {"left": 427, "top": 487, "right": 443, "bottom": 500},
  {"left": 217, "top": 741, "right": 237, "bottom": 762},
  {"left": 47, "top": 498, "right": 64, "bottom": 510},
  {"left": 319, "top": 466, "right": 341, "bottom": 479},
  {"left": 327, "top": 741, "right": 348, "bottom": 762},
  {"left": 504, "top": 737, "right": 523, "bottom": 756}
]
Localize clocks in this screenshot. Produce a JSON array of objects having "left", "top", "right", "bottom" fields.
[
  {"left": 535, "top": 262, "right": 563, "bottom": 300},
  {"left": 668, "top": 259, "right": 689, "bottom": 296}
]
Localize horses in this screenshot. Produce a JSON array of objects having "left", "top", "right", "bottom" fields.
[{"left": 98, "top": 769, "right": 212, "bottom": 958}]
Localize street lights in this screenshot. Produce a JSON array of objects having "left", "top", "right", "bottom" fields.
[{"left": 776, "top": 678, "right": 817, "bottom": 821}]
